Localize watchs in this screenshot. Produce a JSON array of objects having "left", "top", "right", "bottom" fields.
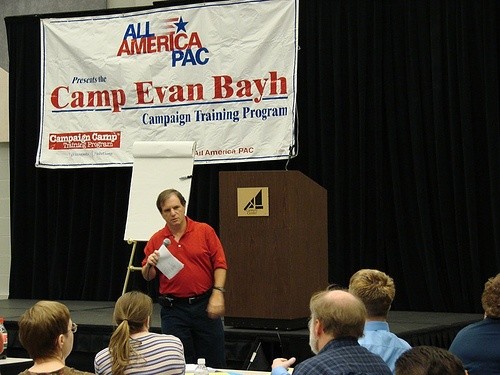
[{"left": 213, "top": 286, "right": 226, "bottom": 294}]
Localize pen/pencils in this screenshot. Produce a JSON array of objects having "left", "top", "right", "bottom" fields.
[{"left": 179, "top": 175, "right": 193, "bottom": 180}]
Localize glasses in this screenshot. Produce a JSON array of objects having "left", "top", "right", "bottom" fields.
[{"left": 63, "top": 323, "right": 78, "bottom": 336}]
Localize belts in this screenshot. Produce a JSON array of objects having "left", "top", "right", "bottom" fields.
[{"left": 165, "top": 288, "right": 212, "bottom": 306}]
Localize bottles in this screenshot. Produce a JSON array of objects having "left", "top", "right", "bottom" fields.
[
  {"left": 194, "top": 358, "right": 209, "bottom": 375},
  {"left": 0, "top": 318, "right": 8, "bottom": 360}
]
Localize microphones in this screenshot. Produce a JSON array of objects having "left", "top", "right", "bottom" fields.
[{"left": 149, "top": 238, "right": 171, "bottom": 268}]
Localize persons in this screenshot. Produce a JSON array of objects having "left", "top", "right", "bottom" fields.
[
  {"left": 394, "top": 345, "right": 469, "bottom": 375},
  {"left": 15, "top": 301, "right": 96, "bottom": 375},
  {"left": 141, "top": 188, "right": 227, "bottom": 370},
  {"left": 446, "top": 271, "right": 499, "bottom": 375},
  {"left": 271, "top": 269, "right": 413, "bottom": 375},
  {"left": 93, "top": 291, "right": 185, "bottom": 375},
  {"left": 292, "top": 289, "right": 395, "bottom": 375}
]
